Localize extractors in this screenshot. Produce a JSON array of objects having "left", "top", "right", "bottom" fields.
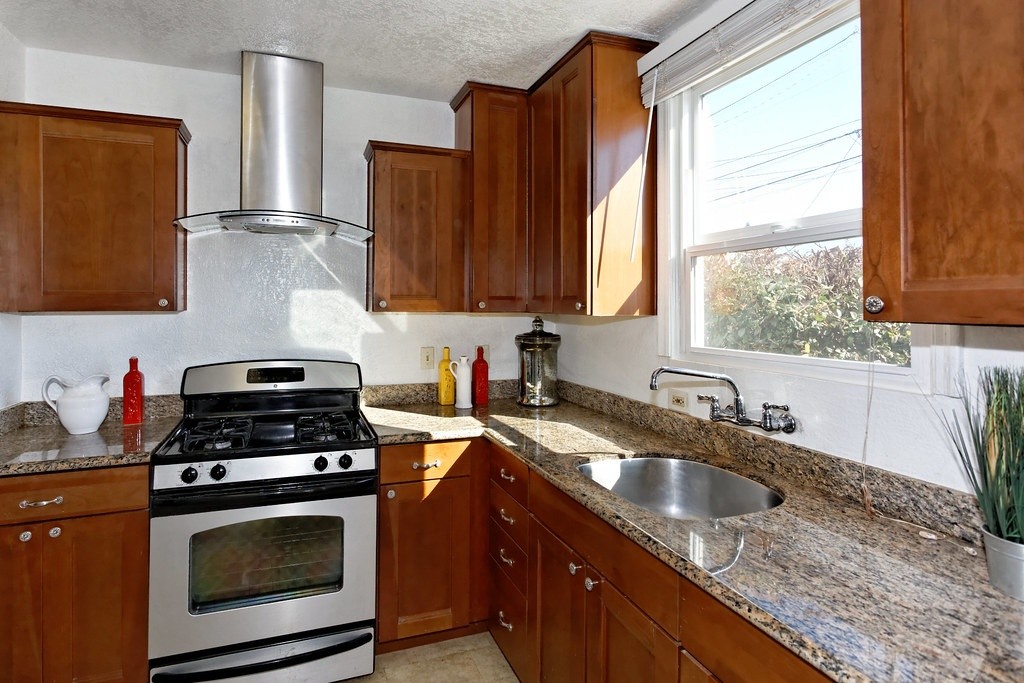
[{"left": 171, "top": 50, "right": 374, "bottom": 242}]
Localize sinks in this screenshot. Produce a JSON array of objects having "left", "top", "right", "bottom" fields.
[{"left": 574, "top": 451, "right": 786, "bottom": 523}]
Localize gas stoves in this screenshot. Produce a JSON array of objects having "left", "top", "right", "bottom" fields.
[{"left": 148, "top": 359, "right": 378, "bottom": 490}]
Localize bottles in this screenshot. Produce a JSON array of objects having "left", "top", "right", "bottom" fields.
[
  {"left": 123, "top": 356, "right": 144, "bottom": 426},
  {"left": 515, "top": 316, "right": 562, "bottom": 407},
  {"left": 472, "top": 347, "right": 489, "bottom": 406},
  {"left": 437, "top": 346, "right": 455, "bottom": 406}
]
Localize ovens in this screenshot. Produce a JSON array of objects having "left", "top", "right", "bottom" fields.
[{"left": 147, "top": 473, "right": 378, "bottom": 683}]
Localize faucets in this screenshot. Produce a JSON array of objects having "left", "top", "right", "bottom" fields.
[{"left": 650, "top": 366, "right": 747, "bottom": 426}]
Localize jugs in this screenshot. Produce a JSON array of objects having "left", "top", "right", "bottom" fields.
[
  {"left": 449, "top": 356, "right": 473, "bottom": 409},
  {"left": 42, "top": 374, "right": 112, "bottom": 435}
]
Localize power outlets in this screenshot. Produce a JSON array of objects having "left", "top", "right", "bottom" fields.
[{"left": 668, "top": 388, "right": 691, "bottom": 413}]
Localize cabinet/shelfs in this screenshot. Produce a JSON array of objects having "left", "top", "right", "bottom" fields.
[
  {"left": 376, "top": 437, "right": 837, "bottom": 683},
  {"left": 859, "top": 1, "right": 1024, "bottom": 328},
  {"left": 0, "top": 101, "right": 192, "bottom": 311},
  {"left": 0, "top": 465, "right": 150, "bottom": 683},
  {"left": 363, "top": 30, "right": 658, "bottom": 317}
]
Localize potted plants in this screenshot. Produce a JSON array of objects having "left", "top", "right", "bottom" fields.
[{"left": 910, "top": 366, "right": 1024, "bottom": 603}]
